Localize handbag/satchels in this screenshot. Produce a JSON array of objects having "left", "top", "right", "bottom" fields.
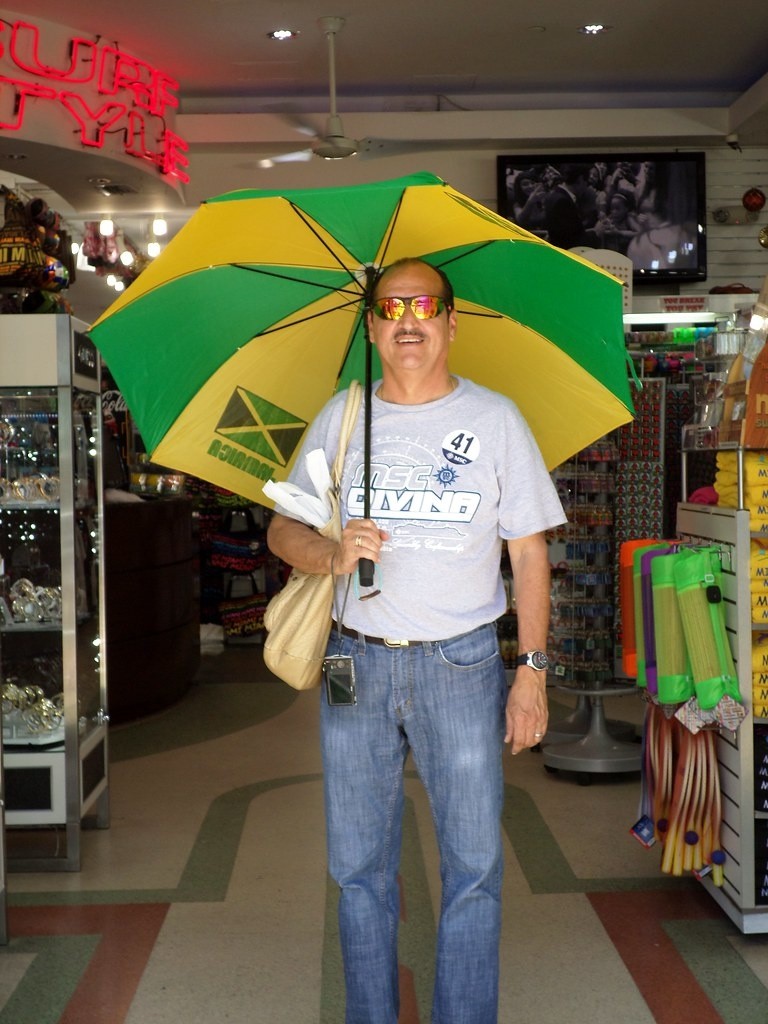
[{"left": 263, "top": 380, "right": 363, "bottom": 691}]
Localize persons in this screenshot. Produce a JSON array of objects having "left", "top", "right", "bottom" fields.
[
  {"left": 267, "top": 257, "right": 571, "bottom": 1023},
  {"left": 513, "top": 161, "right": 692, "bottom": 269}
]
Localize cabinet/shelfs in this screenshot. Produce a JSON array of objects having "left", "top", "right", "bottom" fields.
[{"left": 0, "top": 312, "right": 112, "bottom": 869}]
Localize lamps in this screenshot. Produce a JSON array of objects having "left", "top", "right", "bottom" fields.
[{"left": 70, "top": 213, "right": 169, "bottom": 292}]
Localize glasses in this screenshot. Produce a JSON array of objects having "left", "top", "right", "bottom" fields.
[{"left": 364, "top": 295, "right": 451, "bottom": 321}]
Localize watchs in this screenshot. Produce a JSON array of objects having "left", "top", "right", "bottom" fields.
[{"left": 516, "top": 652, "right": 548, "bottom": 671}]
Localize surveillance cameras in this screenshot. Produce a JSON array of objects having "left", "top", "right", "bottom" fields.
[{"left": 725, "top": 134, "right": 738, "bottom": 147}]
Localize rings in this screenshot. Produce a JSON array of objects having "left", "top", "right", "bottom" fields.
[
  {"left": 535, "top": 733, "right": 542, "bottom": 737},
  {"left": 356, "top": 536, "right": 362, "bottom": 547}
]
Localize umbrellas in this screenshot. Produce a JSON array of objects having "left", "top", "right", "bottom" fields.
[{"left": 83, "top": 170, "right": 635, "bottom": 586}]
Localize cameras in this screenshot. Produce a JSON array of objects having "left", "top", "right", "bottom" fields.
[{"left": 322, "top": 655, "right": 358, "bottom": 706}]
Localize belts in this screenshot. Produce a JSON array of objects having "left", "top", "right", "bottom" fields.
[{"left": 331, "top": 618, "right": 437, "bottom": 649}]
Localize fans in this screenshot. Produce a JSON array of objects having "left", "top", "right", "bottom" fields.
[{"left": 237, "top": 16, "right": 419, "bottom": 172}]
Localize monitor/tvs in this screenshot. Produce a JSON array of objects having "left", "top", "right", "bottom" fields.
[{"left": 496, "top": 151, "right": 708, "bottom": 285}]
